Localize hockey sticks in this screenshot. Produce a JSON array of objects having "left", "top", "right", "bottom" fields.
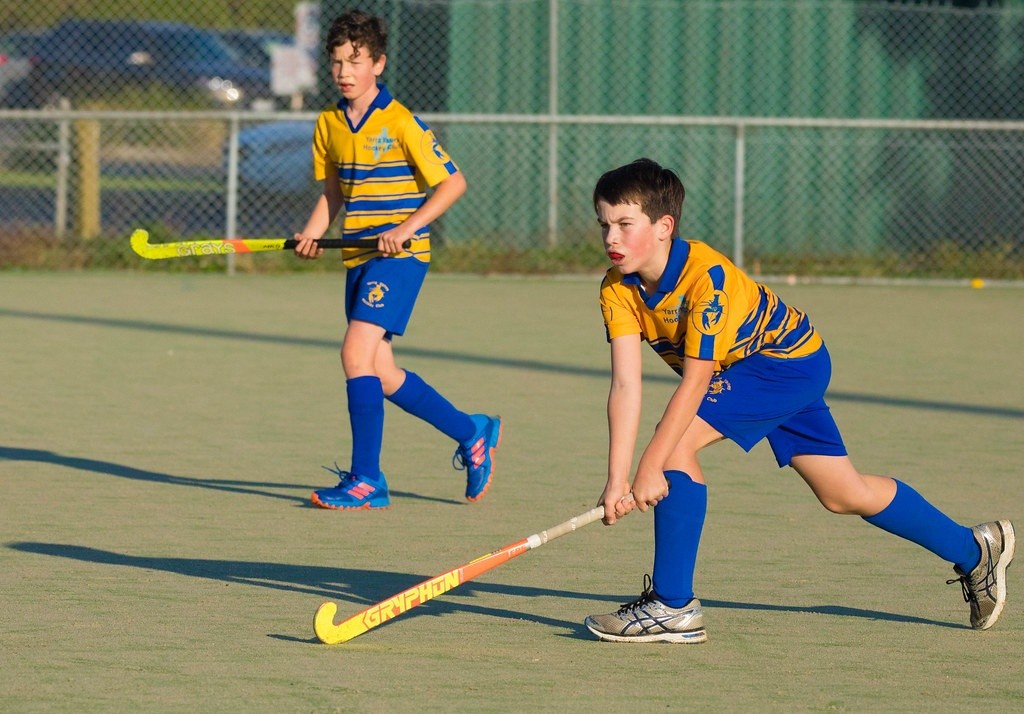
[
  {"left": 129, "top": 227, "right": 413, "bottom": 262},
  {"left": 312, "top": 476, "right": 672, "bottom": 646}
]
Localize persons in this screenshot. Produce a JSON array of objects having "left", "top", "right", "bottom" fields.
[
  {"left": 583, "top": 157, "right": 1015, "bottom": 648},
  {"left": 292, "top": 10, "right": 499, "bottom": 510}
]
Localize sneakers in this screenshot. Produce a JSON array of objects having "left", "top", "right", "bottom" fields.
[
  {"left": 311, "top": 463, "right": 392, "bottom": 510},
  {"left": 452, "top": 414, "right": 503, "bottom": 502},
  {"left": 584, "top": 573, "right": 707, "bottom": 644},
  {"left": 946, "top": 520, "right": 1016, "bottom": 631}
]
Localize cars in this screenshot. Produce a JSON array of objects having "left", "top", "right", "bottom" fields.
[
  {"left": 223, "top": 117, "right": 323, "bottom": 219},
  {"left": 0, "top": 20, "right": 301, "bottom": 151}
]
